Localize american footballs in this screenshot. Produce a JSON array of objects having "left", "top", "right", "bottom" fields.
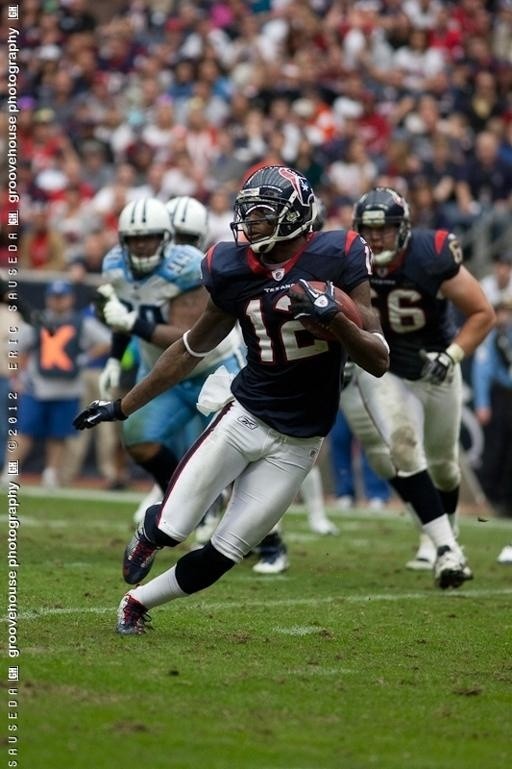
[{"left": 288, "top": 281, "right": 364, "bottom": 342}]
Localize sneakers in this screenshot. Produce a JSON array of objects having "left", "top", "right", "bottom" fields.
[
  {"left": 405, "top": 541, "right": 473, "bottom": 589},
  {"left": 122, "top": 518, "right": 157, "bottom": 585},
  {"left": 195, "top": 489, "right": 290, "bottom": 573},
  {"left": 116, "top": 593, "right": 148, "bottom": 635}
]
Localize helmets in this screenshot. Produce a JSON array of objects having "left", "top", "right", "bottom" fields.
[
  {"left": 116, "top": 198, "right": 175, "bottom": 279},
  {"left": 166, "top": 196, "right": 207, "bottom": 238},
  {"left": 350, "top": 186, "right": 411, "bottom": 267},
  {"left": 228, "top": 164, "right": 324, "bottom": 255}
]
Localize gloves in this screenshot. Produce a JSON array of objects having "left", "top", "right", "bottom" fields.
[
  {"left": 287, "top": 277, "right": 341, "bottom": 331},
  {"left": 422, "top": 342, "right": 464, "bottom": 385},
  {"left": 96, "top": 356, "right": 121, "bottom": 399},
  {"left": 71, "top": 398, "right": 128, "bottom": 431},
  {"left": 101, "top": 292, "right": 138, "bottom": 334}
]
